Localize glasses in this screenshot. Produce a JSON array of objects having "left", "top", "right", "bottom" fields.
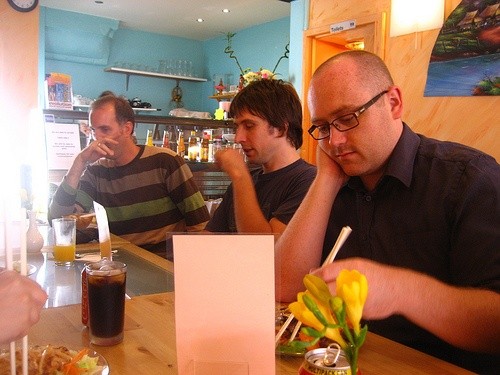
[{"left": 308, "top": 90, "right": 387, "bottom": 140}]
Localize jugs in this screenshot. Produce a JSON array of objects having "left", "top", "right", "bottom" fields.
[{"left": 128, "top": 97, "right": 141, "bottom": 107}]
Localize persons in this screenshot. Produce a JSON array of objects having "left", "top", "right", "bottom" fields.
[
  {"left": 0, "top": 267, "right": 48, "bottom": 344},
  {"left": 201, "top": 78, "right": 317, "bottom": 245},
  {"left": 47, "top": 96, "right": 209, "bottom": 263},
  {"left": 275, "top": 51, "right": 500, "bottom": 375}
]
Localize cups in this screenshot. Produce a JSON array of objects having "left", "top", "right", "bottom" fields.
[
  {"left": 51, "top": 217, "right": 77, "bottom": 266},
  {"left": 73, "top": 94, "right": 95, "bottom": 105},
  {"left": 165, "top": 57, "right": 192, "bottom": 77},
  {"left": 212, "top": 143, "right": 246, "bottom": 163},
  {"left": 85, "top": 260, "right": 127, "bottom": 346}
]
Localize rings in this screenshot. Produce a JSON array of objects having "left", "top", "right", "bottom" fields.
[{"left": 98, "top": 142, "right": 102, "bottom": 146}]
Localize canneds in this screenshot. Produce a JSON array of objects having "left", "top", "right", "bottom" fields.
[
  {"left": 80, "top": 262, "right": 101, "bottom": 327},
  {"left": 298, "top": 343, "right": 361, "bottom": 375}
]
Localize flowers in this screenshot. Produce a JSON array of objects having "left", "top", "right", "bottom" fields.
[
  {"left": 278, "top": 269, "right": 371, "bottom": 375},
  {"left": 222, "top": 30, "right": 292, "bottom": 86}
]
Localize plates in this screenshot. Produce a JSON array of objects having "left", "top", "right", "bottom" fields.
[
  {"left": 274, "top": 349, "right": 306, "bottom": 358},
  {"left": 29, "top": 342, "right": 110, "bottom": 375},
  {"left": 17, "top": 263, "right": 36, "bottom": 275}
]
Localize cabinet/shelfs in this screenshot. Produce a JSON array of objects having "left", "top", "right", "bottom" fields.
[{"left": 40, "top": 108, "right": 262, "bottom": 201}]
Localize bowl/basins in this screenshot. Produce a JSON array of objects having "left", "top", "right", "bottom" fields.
[{"left": 223, "top": 133, "right": 236, "bottom": 142}]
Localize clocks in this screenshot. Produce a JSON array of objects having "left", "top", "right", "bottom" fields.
[{"left": 8, "top": 0, "right": 40, "bottom": 11}]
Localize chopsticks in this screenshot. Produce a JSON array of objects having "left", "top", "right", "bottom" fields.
[
  {"left": 275, "top": 225, "right": 352, "bottom": 347},
  {"left": 2, "top": 207, "right": 29, "bottom": 375}
]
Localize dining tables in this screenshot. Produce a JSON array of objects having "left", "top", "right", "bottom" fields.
[{"left": 0, "top": 197, "right": 475, "bottom": 375}]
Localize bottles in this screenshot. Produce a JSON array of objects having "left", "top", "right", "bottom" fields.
[
  {"left": 188, "top": 131, "right": 196, "bottom": 161},
  {"left": 24, "top": 209, "right": 44, "bottom": 253},
  {"left": 161, "top": 130, "right": 170, "bottom": 148},
  {"left": 176, "top": 130, "right": 185, "bottom": 159},
  {"left": 144, "top": 128, "right": 154, "bottom": 146},
  {"left": 199, "top": 132, "right": 209, "bottom": 162}
]
